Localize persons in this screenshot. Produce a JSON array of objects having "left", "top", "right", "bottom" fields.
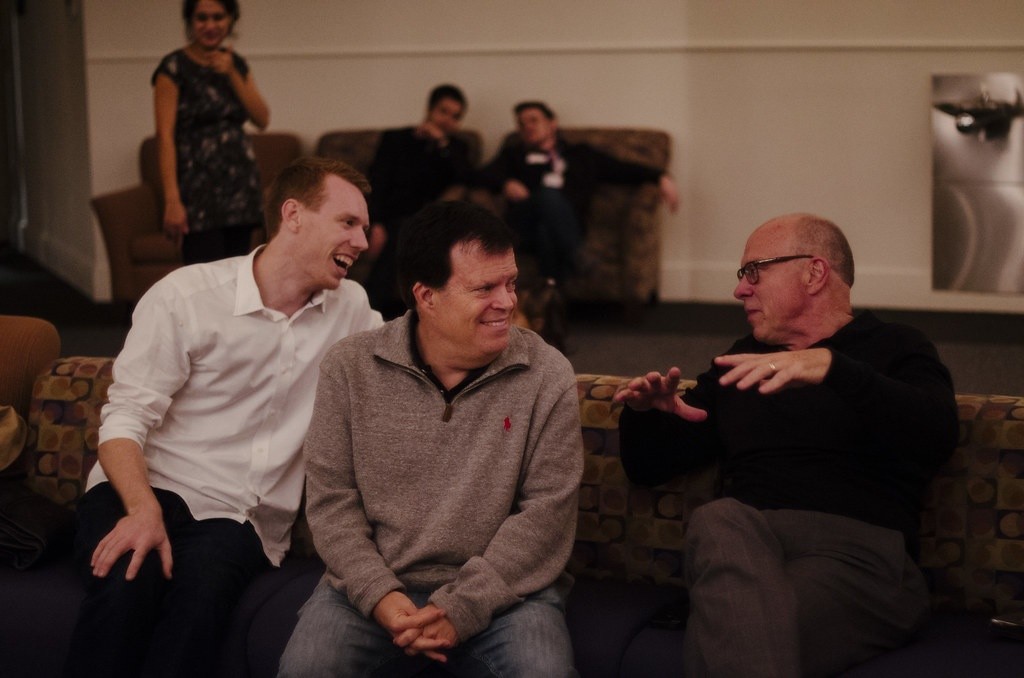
[
  {"left": 474, "top": 101, "right": 680, "bottom": 359},
  {"left": 347, "top": 83, "right": 474, "bottom": 299},
  {"left": 274, "top": 200, "right": 587, "bottom": 678},
  {"left": 150, "top": 0, "right": 269, "bottom": 263},
  {"left": 65, "top": 155, "right": 385, "bottom": 678},
  {"left": 612, "top": 214, "right": 958, "bottom": 678}
]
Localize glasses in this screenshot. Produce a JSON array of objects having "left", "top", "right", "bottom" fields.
[{"left": 736, "top": 255, "right": 813, "bottom": 285}]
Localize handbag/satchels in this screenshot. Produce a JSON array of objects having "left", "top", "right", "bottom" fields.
[{"left": 0, "top": 468, "right": 61, "bottom": 571}]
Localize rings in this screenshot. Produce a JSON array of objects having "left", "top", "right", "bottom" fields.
[{"left": 769, "top": 361, "right": 775, "bottom": 370}]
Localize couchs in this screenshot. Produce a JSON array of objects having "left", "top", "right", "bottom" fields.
[
  {"left": 312, "top": 122, "right": 484, "bottom": 290},
  {"left": 90, "top": 134, "right": 302, "bottom": 309},
  {"left": 493, "top": 120, "right": 673, "bottom": 308},
  {"left": 2, "top": 315, "right": 1024, "bottom": 678}
]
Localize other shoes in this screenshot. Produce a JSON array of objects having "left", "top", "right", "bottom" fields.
[{"left": 531, "top": 284, "right": 565, "bottom": 346}]
